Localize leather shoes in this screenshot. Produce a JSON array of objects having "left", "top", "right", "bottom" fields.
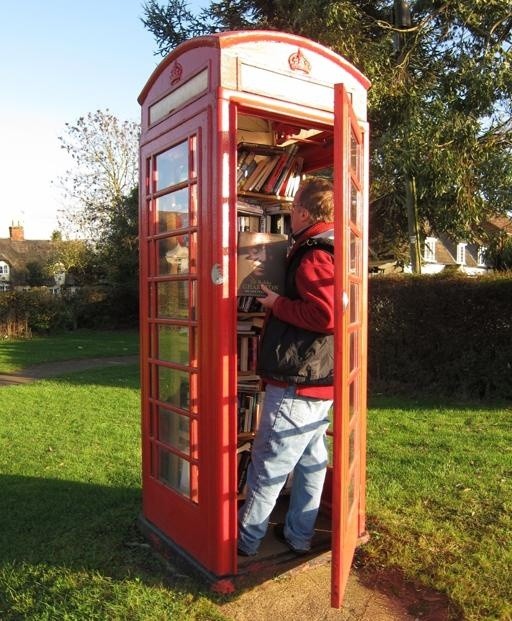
[{"left": 275, "top": 522, "right": 305, "bottom": 554}]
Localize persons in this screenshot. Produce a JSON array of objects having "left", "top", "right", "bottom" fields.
[
  {"left": 242, "top": 245, "right": 274, "bottom": 279},
  {"left": 239, "top": 177, "right": 334, "bottom": 555}
]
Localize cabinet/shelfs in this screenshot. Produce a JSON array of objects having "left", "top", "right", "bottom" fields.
[{"left": 166, "top": 129, "right": 308, "bottom": 511}]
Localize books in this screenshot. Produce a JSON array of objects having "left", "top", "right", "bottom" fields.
[
  {"left": 240, "top": 199, "right": 295, "bottom": 247},
  {"left": 237, "top": 378, "right": 267, "bottom": 436},
  {"left": 237, "top": 111, "right": 303, "bottom": 197},
  {"left": 237, "top": 446, "right": 255, "bottom": 500},
  {"left": 238, "top": 232, "right": 290, "bottom": 299},
  {"left": 235, "top": 298, "right": 272, "bottom": 376}
]
